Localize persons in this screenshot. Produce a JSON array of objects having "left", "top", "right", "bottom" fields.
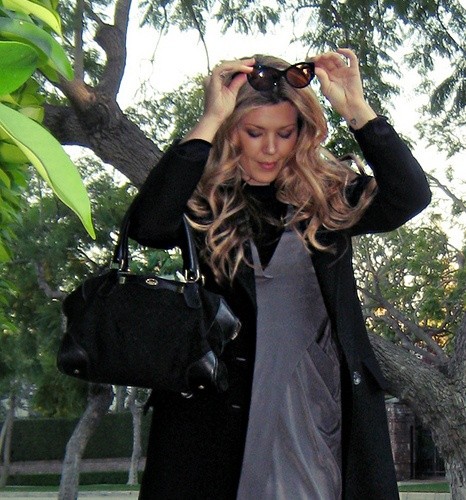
[{"left": 127, "top": 46, "right": 432, "bottom": 500}]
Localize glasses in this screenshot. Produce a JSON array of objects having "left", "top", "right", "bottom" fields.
[{"left": 231, "top": 60, "right": 317, "bottom": 92}]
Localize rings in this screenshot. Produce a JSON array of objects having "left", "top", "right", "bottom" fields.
[{"left": 219, "top": 72, "right": 226, "bottom": 78}]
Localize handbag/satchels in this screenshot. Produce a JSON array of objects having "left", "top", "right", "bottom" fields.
[{"left": 58, "top": 215, "right": 245, "bottom": 399}]
